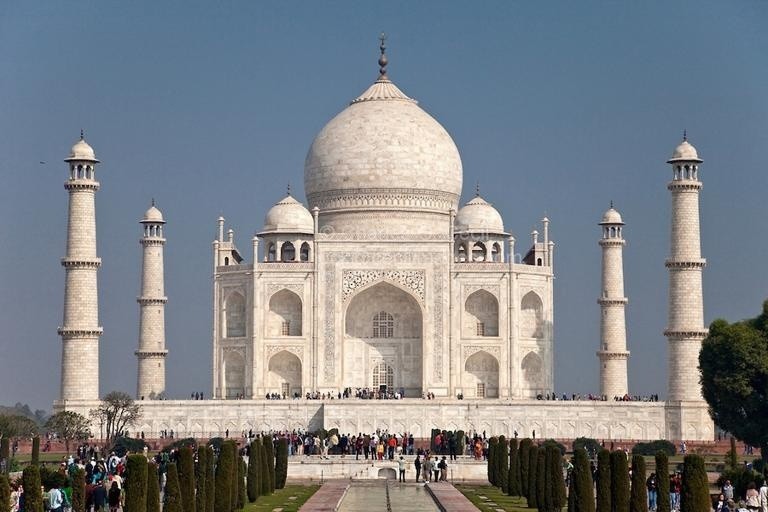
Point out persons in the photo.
[431,392,435,399]
[433,456,440,482]
[191,392,195,399]
[196,392,199,399]
[236,393,239,400]
[0,429,178,512]
[460,393,463,399]
[240,428,414,460]
[414,456,422,482]
[423,458,432,481]
[441,456,448,482]
[457,393,460,400]
[426,391,432,399]
[265,386,405,400]
[200,392,203,400]
[397,456,406,483]
[536,392,659,402]
[422,457,434,483]
[434,430,490,461]
[240,394,244,400]
[562,432,768,512]
[226,429,229,437]
